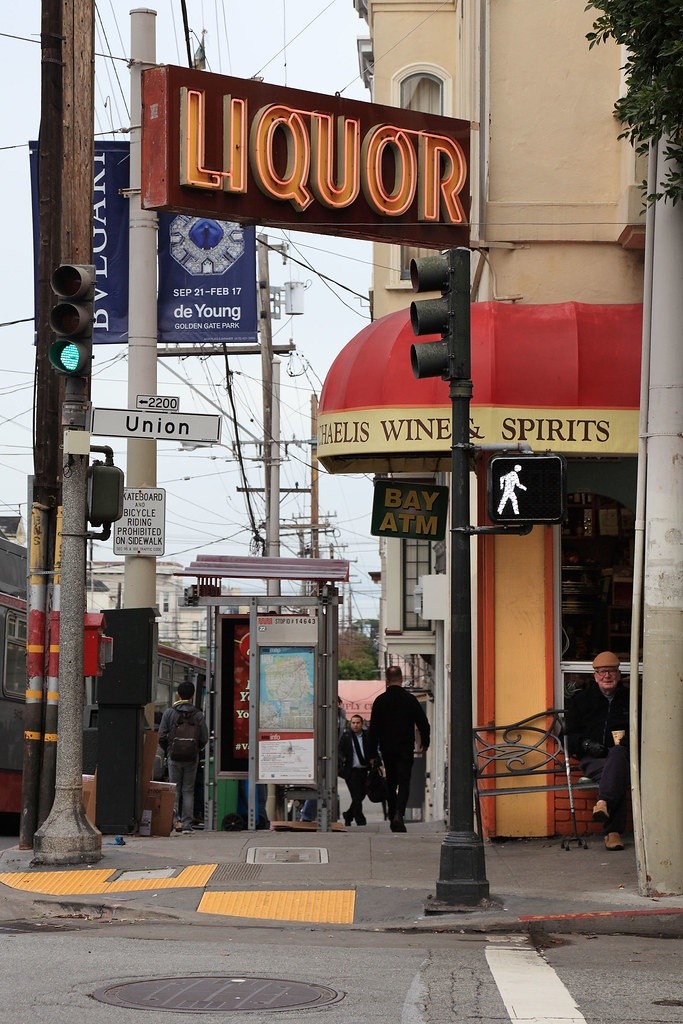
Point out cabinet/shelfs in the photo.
[561,493,643,663]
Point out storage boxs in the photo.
[80,731,177,838]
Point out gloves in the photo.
[582,739,609,758]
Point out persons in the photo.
[159,680,208,831]
[566,650,641,850]
[369,666,431,832]
[338,714,368,828]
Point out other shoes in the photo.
[605,832,624,850]
[389,813,406,832]
[594,804,609,823]
[176,822,182,831]
[181,823,194,833]
[342,812,352,827]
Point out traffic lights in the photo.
[48,263,98,379]
[407,250,457,383]
[489,453,567,524]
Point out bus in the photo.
[0,591,214,815]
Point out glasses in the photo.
[595,670,618,675]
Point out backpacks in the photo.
[168,707,201,763]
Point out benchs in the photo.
[471,710,600,845]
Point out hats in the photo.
[592,652,620,668]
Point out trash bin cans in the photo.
[201,758,268,833]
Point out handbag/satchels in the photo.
[367,765,388,803]
